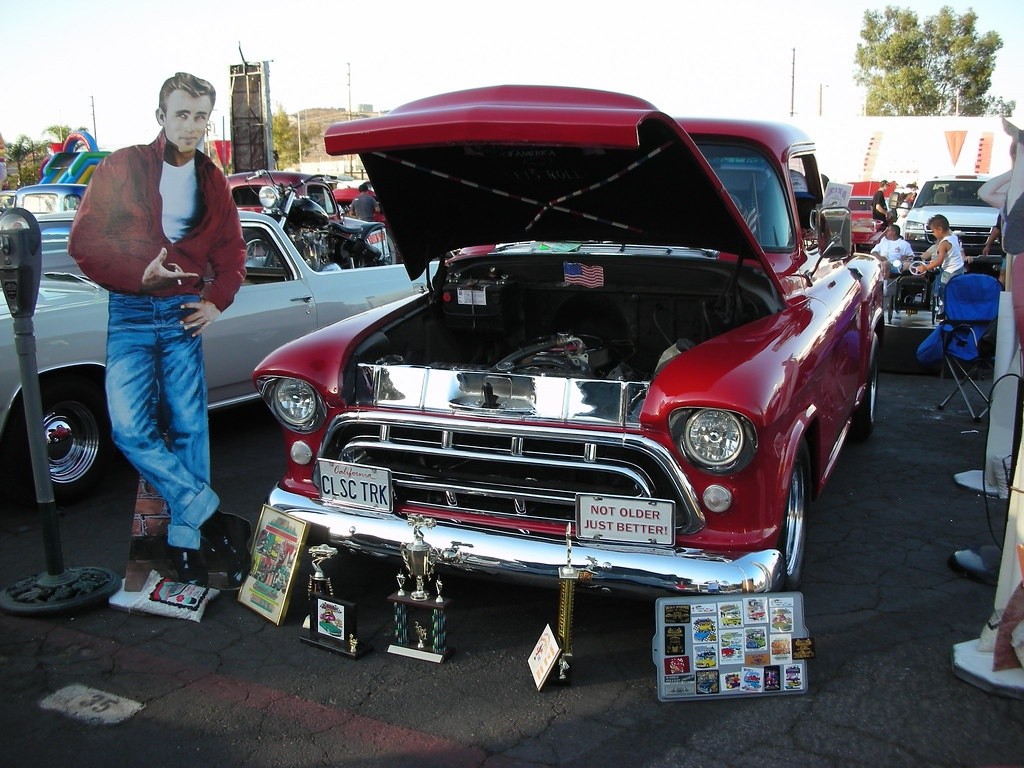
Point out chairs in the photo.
[915,273,1004,424]
[933,191,953,205]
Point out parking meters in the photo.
[0,207,122,615]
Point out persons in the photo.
[873,180,889,232]
[983,213,1006,283]
[350,184,380,222]
[916,214,973,322]
[907,186,917,209]
[870,224,915,274]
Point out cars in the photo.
[0,166,442,499]
[253,83,886,603]
[841,180,899,244]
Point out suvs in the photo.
[903,175,1004,268]
